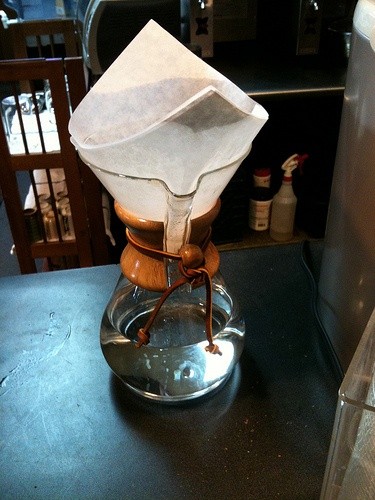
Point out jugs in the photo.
[78,143,252,402]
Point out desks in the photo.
[0,238,342,500]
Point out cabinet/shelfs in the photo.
[0,19,111,275]
[203,38,347,252]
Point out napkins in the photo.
[67,19,270,223]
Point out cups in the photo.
[22,190,76,243]
[1,75,73,154]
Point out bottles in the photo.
[249,169,273,241]
[320,308,375,500]
[270,154,308,241]
